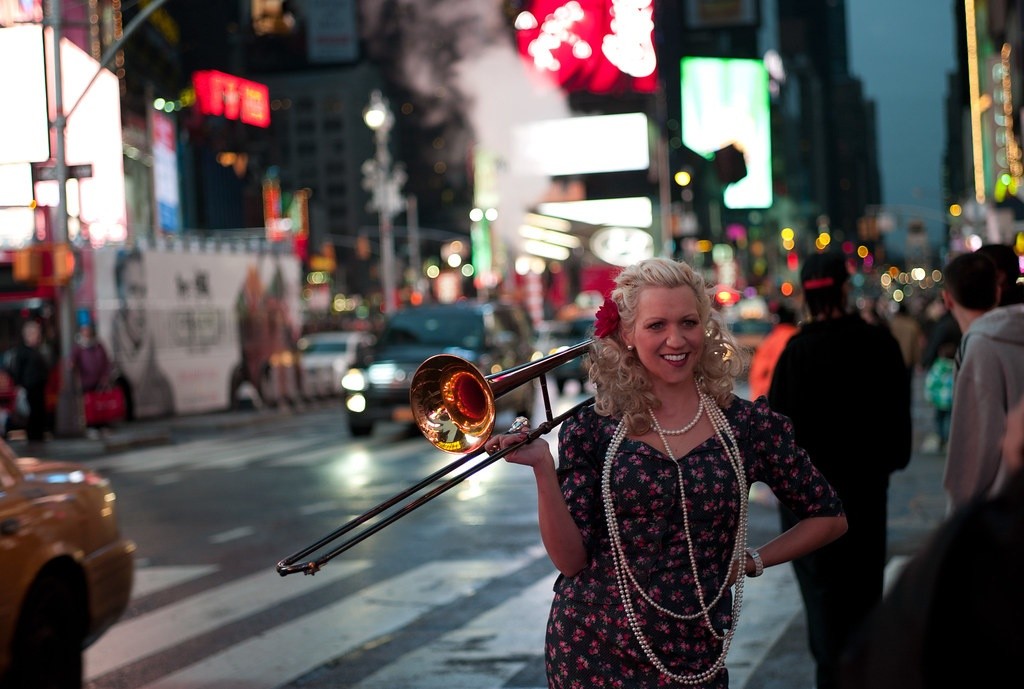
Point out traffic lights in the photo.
[713,285,735,309]
[504,0,657,117]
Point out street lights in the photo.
[363,92,408,310]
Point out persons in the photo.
[749,247,1024,689]
[483,257,848,689]
[7,319,51,449]
[108,249,176,415]
[67,323,112,439]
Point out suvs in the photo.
[549,315,595,393]
[342,299,541,438]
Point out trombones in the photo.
[273,339,595,577]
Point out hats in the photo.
[801,254,844,299]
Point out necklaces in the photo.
[602,379,749,684]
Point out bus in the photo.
[0,235,305,438]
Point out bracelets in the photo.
[745,547,764,578]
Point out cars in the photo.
[302,333,366,368]
[0,436,136,689]
